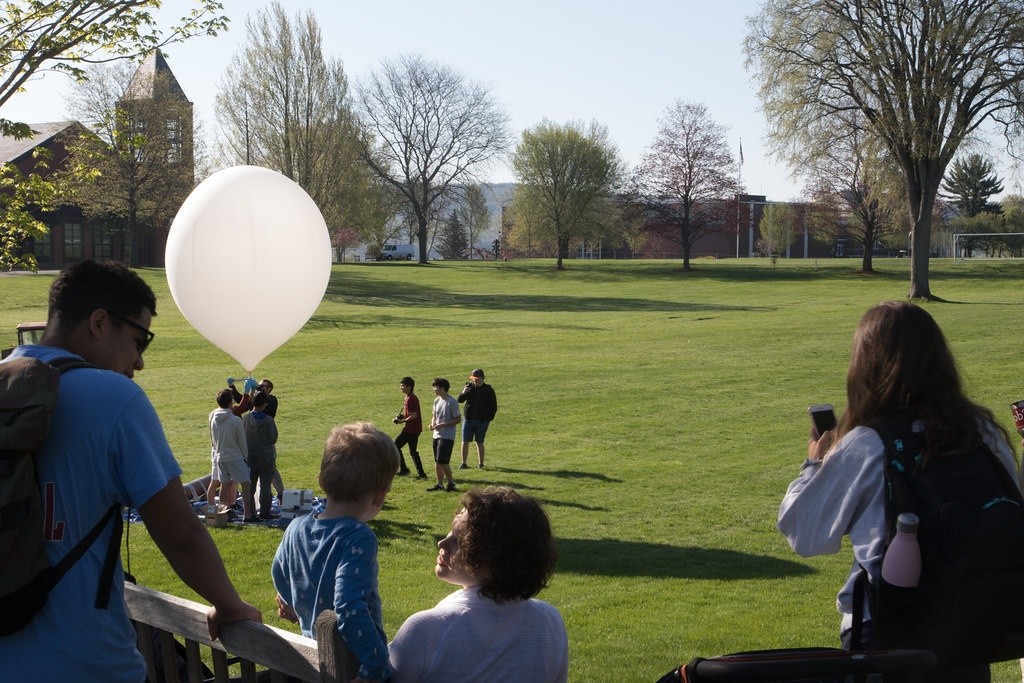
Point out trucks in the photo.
[380,243,415,261]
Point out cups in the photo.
[1010,399,1024,428]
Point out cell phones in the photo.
[808,403,837,437]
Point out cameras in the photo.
[393,414,405,424]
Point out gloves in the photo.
[228,378,235,385]
[244,377,258,394]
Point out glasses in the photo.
[260,384,267,387]
[89,309,155,355]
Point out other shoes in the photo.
[205,502,279,522]
[277,490,283,505]
[396,468,410,476]
[427,485,444,491]
[413,473,426,478]
[460,464,467,469]
[478,464,484,468]
[445,484,455,491]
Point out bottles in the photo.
[881,512,922,588]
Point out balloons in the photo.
[165,165,332,373]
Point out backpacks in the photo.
[857,405,1024,663]
[0,347,123,638]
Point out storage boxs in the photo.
[281,489,313,519]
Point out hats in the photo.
[469,369,484,380]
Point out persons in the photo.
[270,421,401,683]
[392,377,427,479]
[775,300,1024,683]
[387,486,569,683]
[426,377,461,492]
[207,389,234,505]
[242,392,280,519]
[0,259,263,683]
[457,368,497,469]
[210,391,264,522]
[227,377,284,505]
